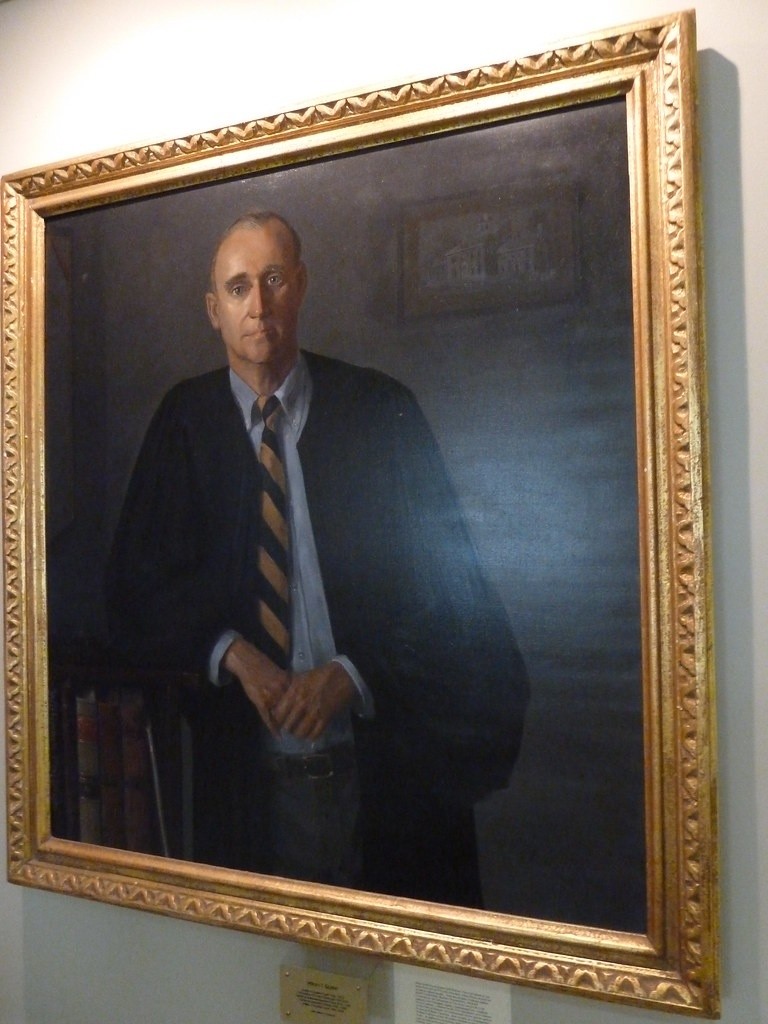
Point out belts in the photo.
[263,743,357,779]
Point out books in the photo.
[55,680,147,851]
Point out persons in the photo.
[104,216,531,909]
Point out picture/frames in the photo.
[2,8,726,1016]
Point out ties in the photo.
[255,395,293,668]
